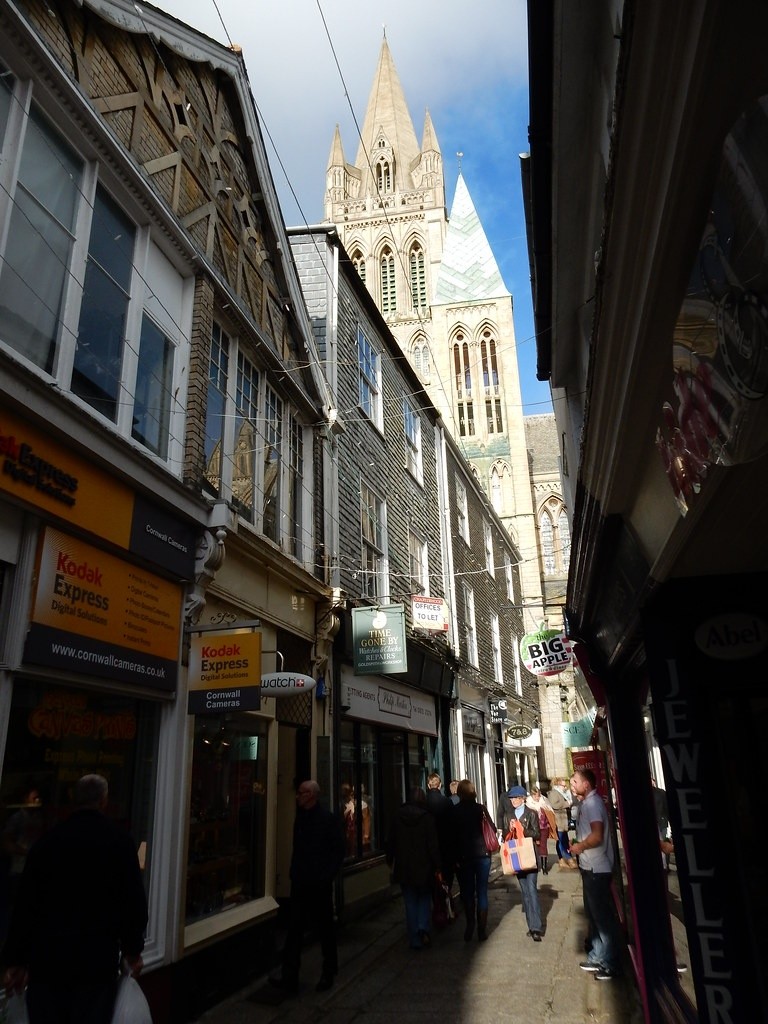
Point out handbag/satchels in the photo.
[500,820,537,876]
[432,872,458,928]
[111,959,153,1024]
[480,805,500,852]
[0,987,30,1024]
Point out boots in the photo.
[541,857,548,875]
[477,904,489,940]
[464,898,475,941]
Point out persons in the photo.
[567,768,624,981]
[389,784,441,953]
[502,786,545,942]
[426,773,460,922]
[3,774,149,1024]
[526,777,579,875]
[280,780,346,995]
[661,819,687,974]
[5,789,48,877]
[449,780,497,943]
[186,841,224,921]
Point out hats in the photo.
[508,786,527,797]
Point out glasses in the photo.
[297,791,311,795]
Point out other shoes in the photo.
[594,969,612,979]
[677,964,688,972]
[315,966,339,992]
[558,858,579,869]
[580,962,602,971]
[527,930,541,941]
[408,929,432,949]
